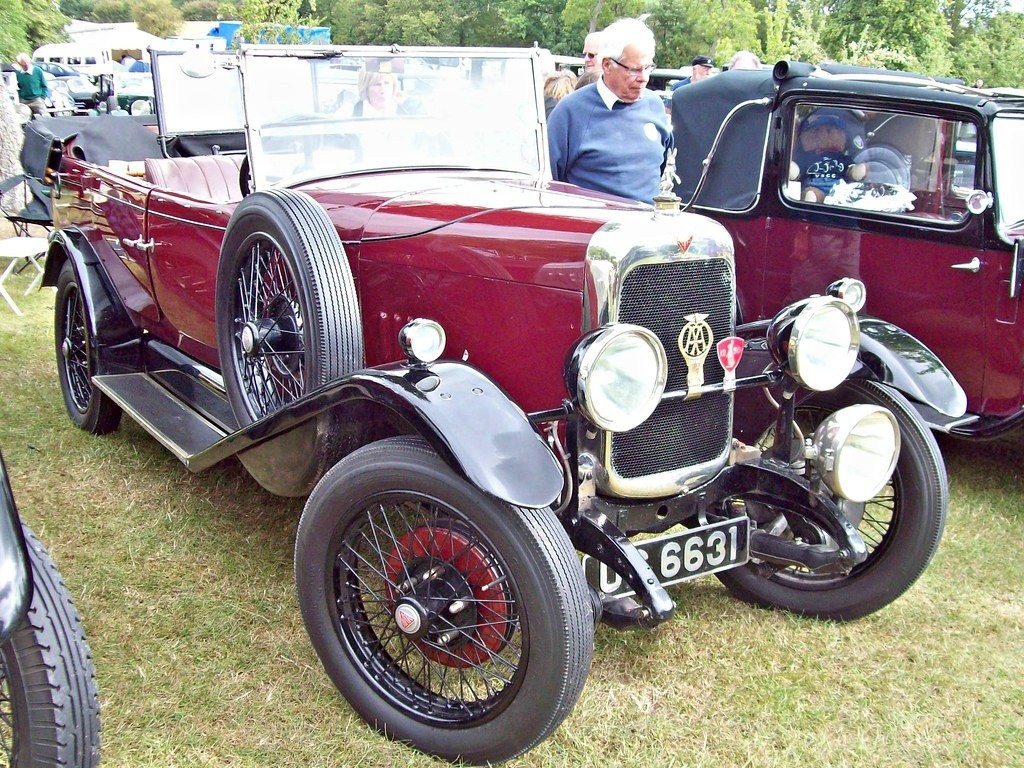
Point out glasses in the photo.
[582,52,598,58]
[608,57,657,76]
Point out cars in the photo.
[668,59,1024,437]
[19,39,967,766]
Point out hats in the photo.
[692,55,714,68]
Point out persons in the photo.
[544,18,763,209]
[15,53,52,129]
[352,63,407,117]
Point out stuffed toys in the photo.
[789,107,868,203]
